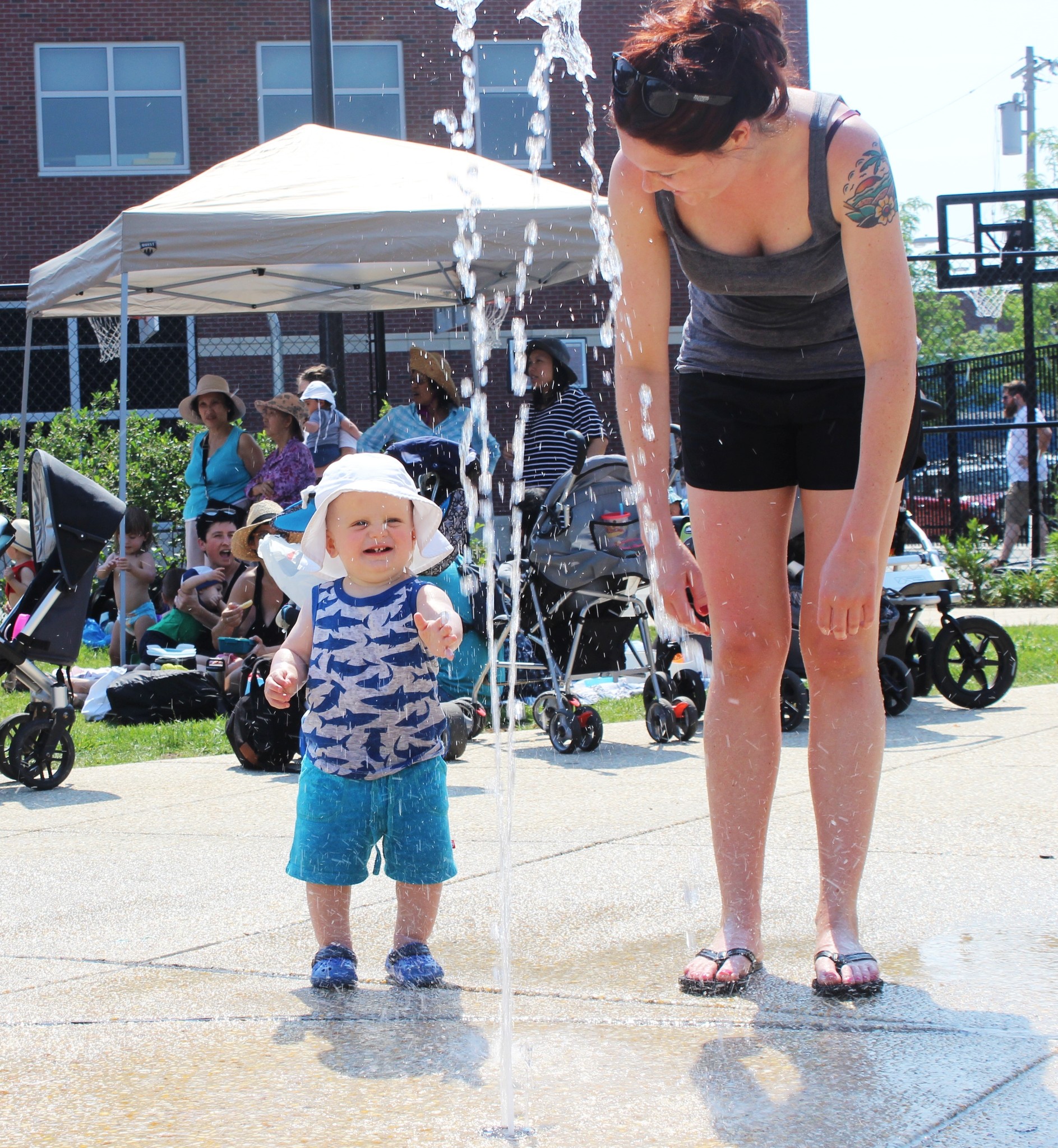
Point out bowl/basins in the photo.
[217,637,255,654]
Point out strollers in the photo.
[640,389,1020,733]
[445,422,705,751]
[0,447,141,790]
[227,434,480,771]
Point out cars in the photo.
[905,464,1008,538]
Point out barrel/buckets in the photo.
[154,656,197,671]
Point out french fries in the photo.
[237,599,253,609]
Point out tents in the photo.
[19,120,610,671]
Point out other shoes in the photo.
[385,942,443,990]
[307,945,360,990]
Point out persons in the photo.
[357,347,501,476]
[265,452,464,996]
[98,503,157,667]
[3,518,34,689]
[70,508,250,709]
[605,1,921,994]
[178,375,266,569]
[983,380,1052,572]
[137,565,226,667]
[295,365,358,457]
[212,502,300,696]
[245,392,314,511]
[504,337,608,488]
[157,567,188,624]
[299,379,362,486]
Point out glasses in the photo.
[202,509,237,517]
[250,530,290,543]
[610,50,734,119]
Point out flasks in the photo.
[205,657,225,691]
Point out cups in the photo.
[601,511,631,548]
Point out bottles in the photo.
[12,613,30,641]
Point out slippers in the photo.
[678,948,763,1000]
[812,951,882,1000]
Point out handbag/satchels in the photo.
[203,496,246,530]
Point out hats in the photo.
[525,338,578,387]
[178,374,246,426]
[181,566,227,592]
[8,518,34,556]
[253,392,311,443]
[408,346,462,410]
[300,380,336,412]
[232,499,304,562]
[297,452,455,580]
